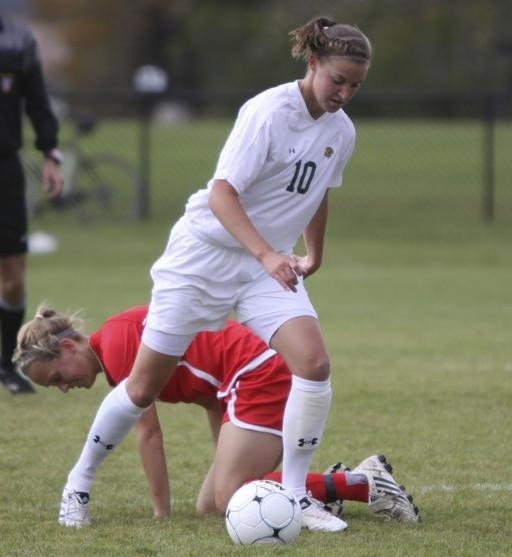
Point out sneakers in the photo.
[0,369,35,393]
[319,462,350,517]
[58,485,93,529]
[351,453,423,524]
[295,494,348,533]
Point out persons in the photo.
[58,18,371,532]
[13,305,423,523]
[0,10,74,389]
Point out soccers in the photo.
[225,480,303,546]
